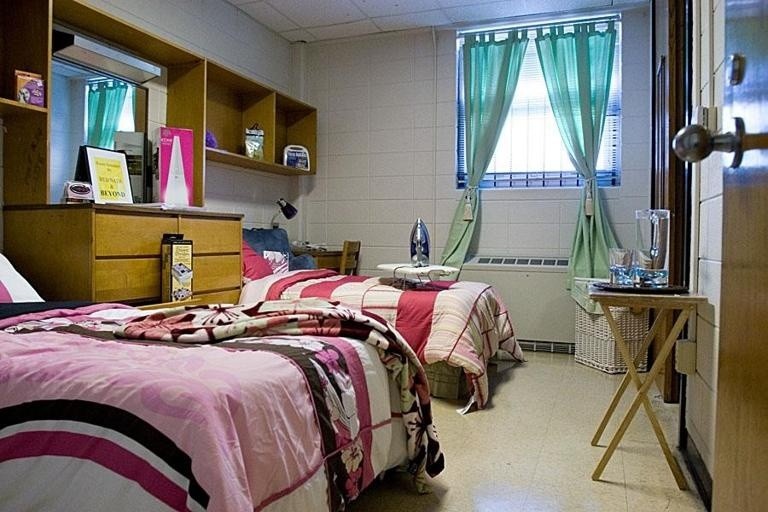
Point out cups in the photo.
[634,209,671,290]
[606,247,635,288]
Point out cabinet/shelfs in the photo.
[0,0,208,207]
[205,58,318,176]
[0,204,245,312]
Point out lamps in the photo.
[271,197,298,229]
[52,29,162,87]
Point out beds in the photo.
[0,252,450,511]
[239,228,528,414]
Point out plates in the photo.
[594,281,690,295]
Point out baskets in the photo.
[574,306,650,376]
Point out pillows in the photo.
[263,251,289,274]
[290,252,318,271]
[243,237,274,283]
[243,228,290,253]
[0,252,45,304]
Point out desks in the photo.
[290,247,342,270]
[589,285,708,491]
[376,261,460,292]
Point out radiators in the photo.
[457,256,581,355]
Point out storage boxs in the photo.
[112,130,145,204]
[148,126,194,210]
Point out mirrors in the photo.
[51,56,149,203]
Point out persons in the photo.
[18,87,31,104]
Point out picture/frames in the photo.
[83,144,136,205]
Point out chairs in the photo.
[339,239,362,276]
